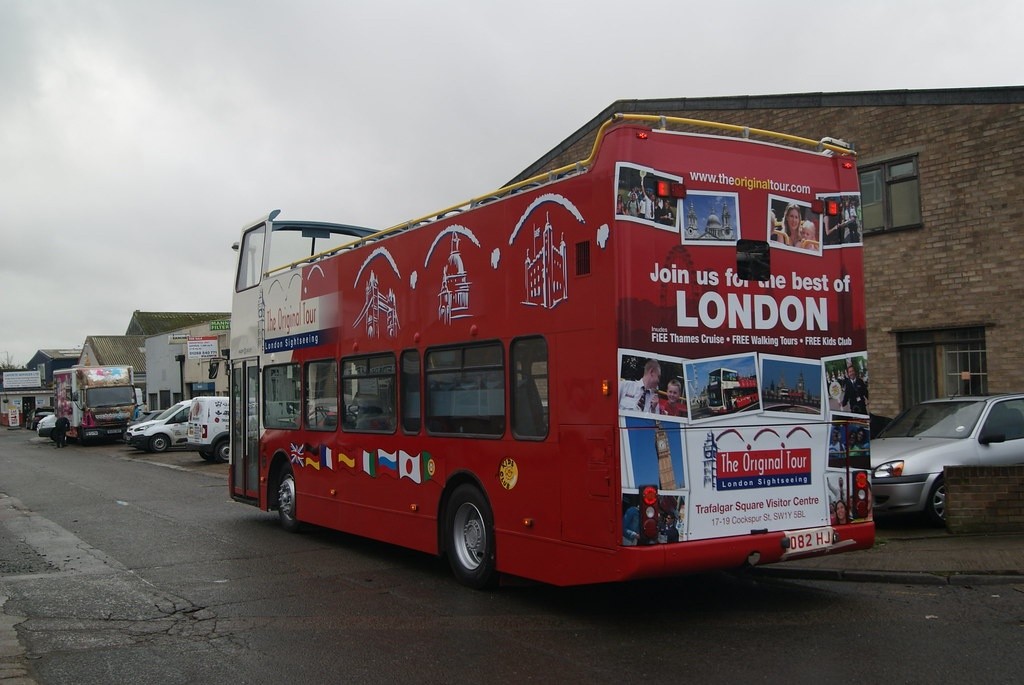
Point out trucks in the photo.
[53,366,137,444]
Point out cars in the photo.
[869,393,1024,522]
[126,411,164,429]
[36,414,58,443]
[26,406,55,430]
[187,397,261,461]
[291,394,365,430]
[870,411,894,442]
[126,398,193,451]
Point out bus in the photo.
[780,386,804,398]
[708,366,758,414]
[229,112,875,588]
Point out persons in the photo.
[843,430,860,460]
[851,481,872,524]
[863,420,870,446]
[853,424,869,458]
[351,397,377,431]
[847,493,854,520]
[839,365,869,415]
[616,170,675,227]
[848,201,860,230]
[618,357,663,416]
[844,199,850,225]
[824,425,846,461]
[622,500,644,546]
[662,513,681,545]
[779,203,809,249]
[791,220,820,251]
[54,411,73,449]
[770,208,787,248]
[835,500,852,523]
[822,200,842,247]
[656,377,689,421]
[832,499,837,510]
[829,503,836,524]
[825,472,852,507]
